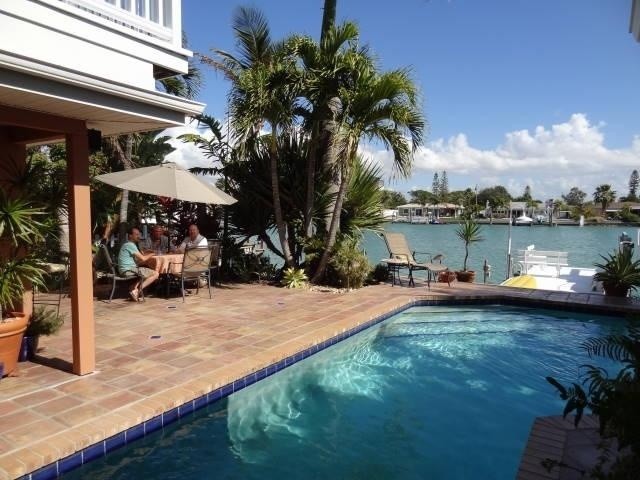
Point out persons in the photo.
[117,227,159,303]
[176,224,208,295]
[145,226,176,292]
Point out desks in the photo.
[32,263,66,317]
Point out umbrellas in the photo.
[93,161,239,296]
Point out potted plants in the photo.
[0,186,69,380]
[453,216,481,283]
[17,304,65,362]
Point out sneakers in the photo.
[128,289,138,304]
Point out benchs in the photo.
[517,250,568,277]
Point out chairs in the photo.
[99,238,223,303]
[379,232,451,289]
